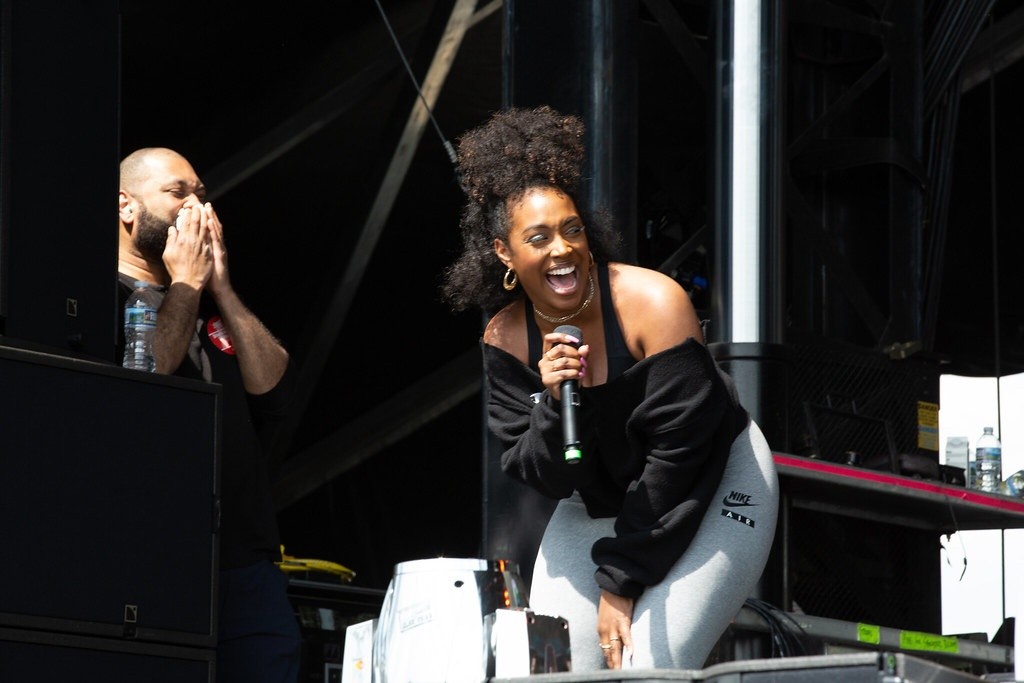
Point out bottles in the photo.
[1000,478,1024,495]
[122,282,158,372]
[975,427,1001,493]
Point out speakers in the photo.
[0,0,222,683]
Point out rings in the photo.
[609,636,620,641]
[599,643,612,649]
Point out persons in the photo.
[118,146,306,683]
[437,105,786,683]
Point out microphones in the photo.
[552,326,584,463]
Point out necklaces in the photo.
[532,270,596,324]
